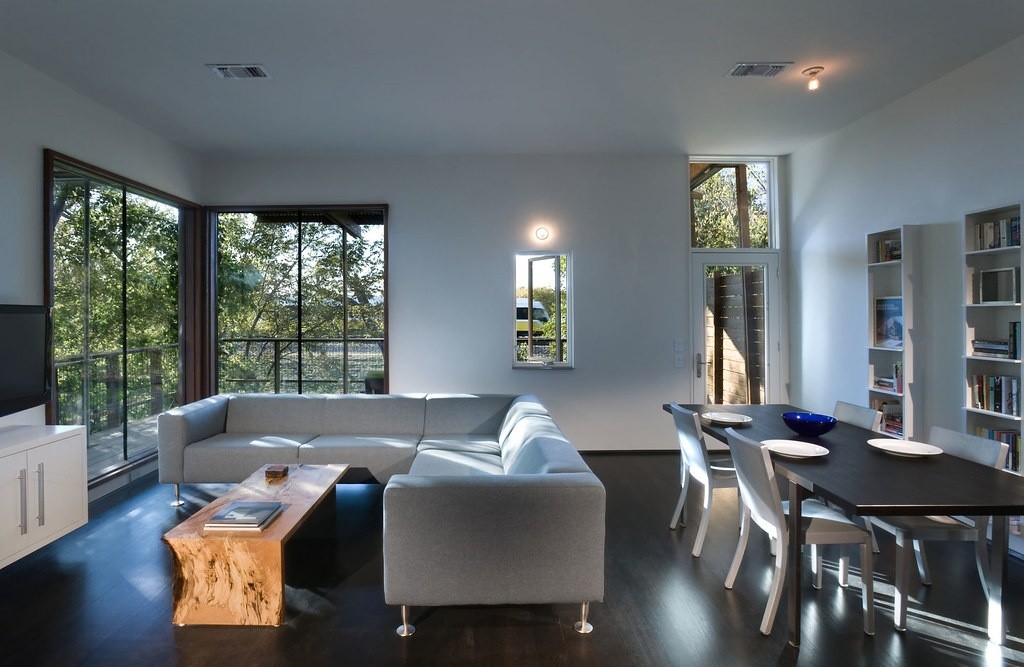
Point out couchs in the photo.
[157,392,606,638]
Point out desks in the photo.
[663,404,1024,647]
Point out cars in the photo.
[516,297,549,338]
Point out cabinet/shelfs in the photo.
[0,424,88,570]
[864,225,923,442]
[963,200,1024,555]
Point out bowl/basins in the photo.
[782,412,837,437]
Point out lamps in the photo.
[802,66,825,92]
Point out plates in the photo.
[701,412,754,425]
[866,438,944,457]
[760,439,830,458]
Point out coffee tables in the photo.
[160,463,350,627]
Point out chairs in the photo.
[669,401,740,557]
[839,425,1008,631]
[725,427,874,634]
[805,401,883,553]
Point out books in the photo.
[874,240,902,262]
[874,361,903,393]
[973,425,1021,473]
[975,216,1021,251]
[204,501,282,527]
[870,398,904,436]
[972,374,1021,416]
[202,501,284,533]
[971,321,1021,360]
